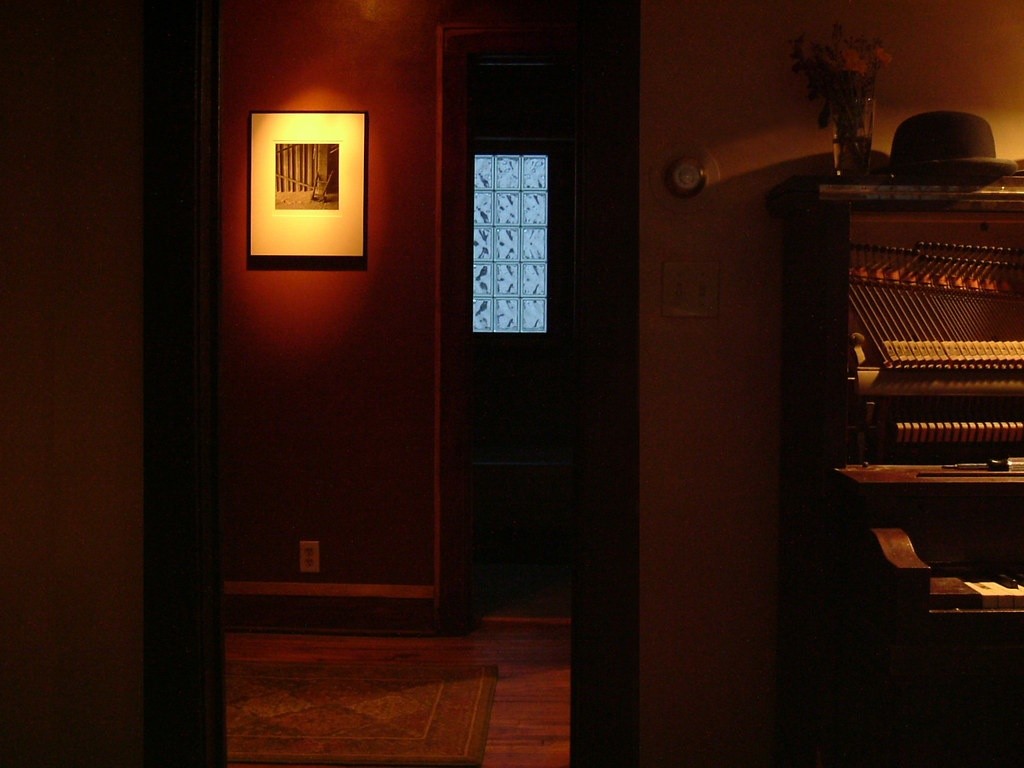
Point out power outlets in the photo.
[299,541,321,573]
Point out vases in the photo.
[830,96,876,185]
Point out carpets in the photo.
[216,657,499,768]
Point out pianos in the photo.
[763,170,1024,768]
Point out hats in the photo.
[876,111,1018,175]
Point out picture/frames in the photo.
[246,109,370,261]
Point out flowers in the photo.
[787,21,894,159]
